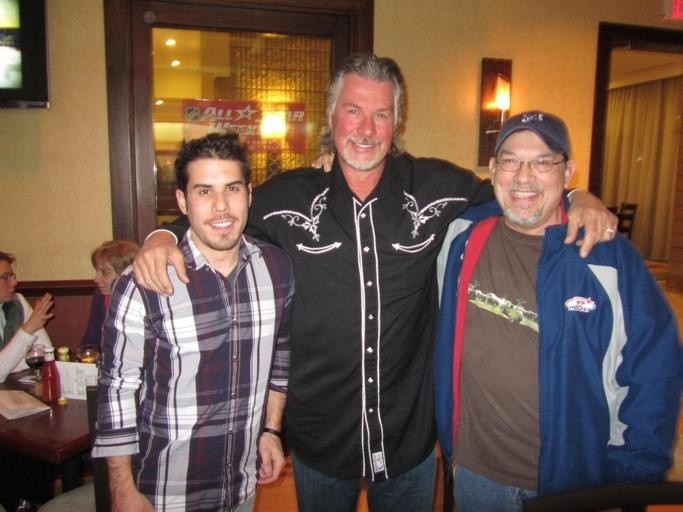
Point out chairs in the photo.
[38,385,113,512]
[616,201,638,240]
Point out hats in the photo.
[495,113,570,159]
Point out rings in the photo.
[605,227,615,234]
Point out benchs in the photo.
[13,280,101,354]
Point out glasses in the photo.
[496,158,564,174]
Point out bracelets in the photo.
[262,425,284,439]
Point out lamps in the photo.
[477,57,513,167]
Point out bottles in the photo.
[57,346,70,362]
[41,345,60,403]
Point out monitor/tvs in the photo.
[0,0,51,108]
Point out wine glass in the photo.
[25,343,46,381]
[74,343,98,364]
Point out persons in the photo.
[0,249,56,384]
[434,103,683,511]
[80,238,142,352]
[130,49,626,512]
[91,130,298,512]
